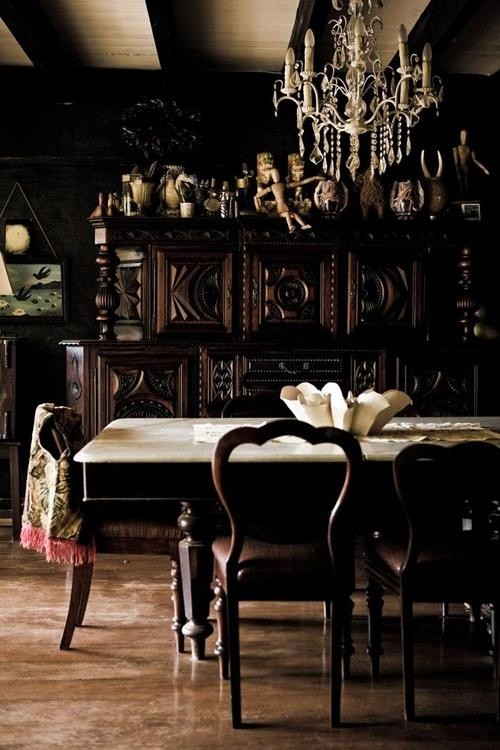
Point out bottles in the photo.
[394,181,419,220]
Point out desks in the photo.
[74,413,500,506]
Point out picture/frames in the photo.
[0,257,69,324]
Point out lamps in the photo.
[270,0,447,182]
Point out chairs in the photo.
[209,405,363,672]
[18,397,193,655]
[0,340,22,547]
[362,446,500,669]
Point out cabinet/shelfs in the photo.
[60,214,500,442]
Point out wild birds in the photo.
[13,285,33,302]
[32,265,52,281]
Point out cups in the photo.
[179,202,194,218]
